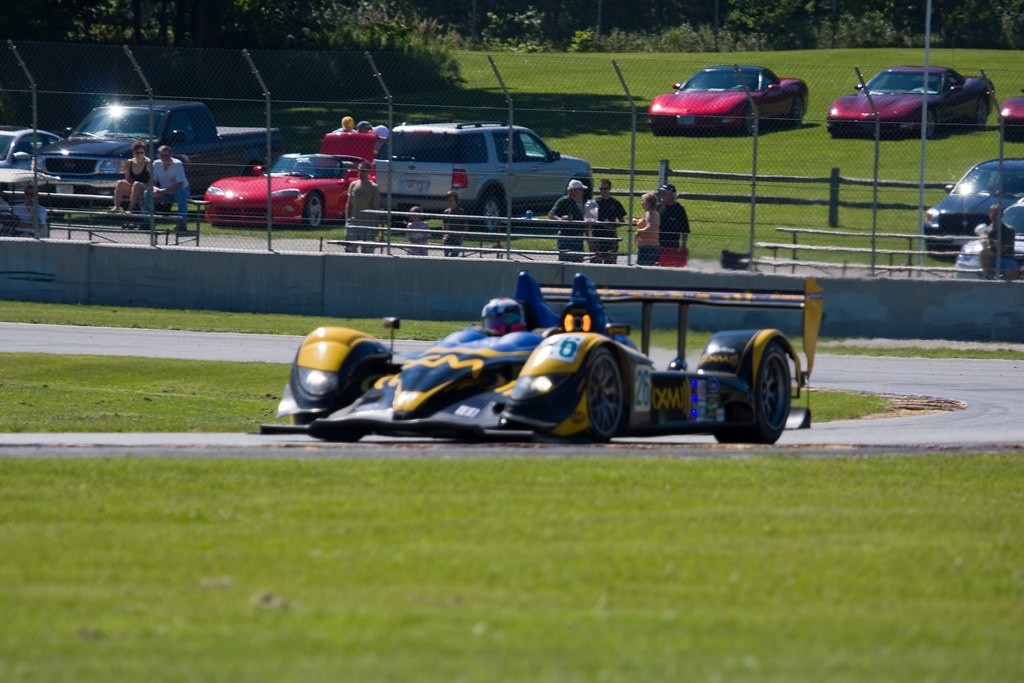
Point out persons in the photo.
[584,179,627,264]
[139,145,191,231]
[548,177,587,263]
[979,205,1018,281]
[333,116,389,151]
[633,184,690,268]
[405,206,432,255]
[474,297,526,336]
[344,162,379,254]
[0,185,48,238]
[443,190,468,257]
[110,141,152,214]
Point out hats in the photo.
[341,116,354,128]
[567,179,588,190]
[357,121,373,130]
[372,125,389,139]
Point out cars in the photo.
[370,120,594,235]
[953,198,1024,280]
[924,157,1024,261]
[0,125,66,221]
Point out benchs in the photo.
[0,189,211,247]
[738,226,1024,280]
[327,208,635,264]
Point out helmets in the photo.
[482,298,527,336]
[657,184,676,194]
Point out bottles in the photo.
[583,199,598,221]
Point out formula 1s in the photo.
[259,270,824,445]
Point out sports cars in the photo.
[826,65,995,139]
[998,88,1024,143]
[204,132,378,231]
[647,65,809,137]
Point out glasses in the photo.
[654,193,667,197]
[599,187,609,191]
[356,169,367,172]
[133,151,143,154]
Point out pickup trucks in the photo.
[31,99,285,220]
